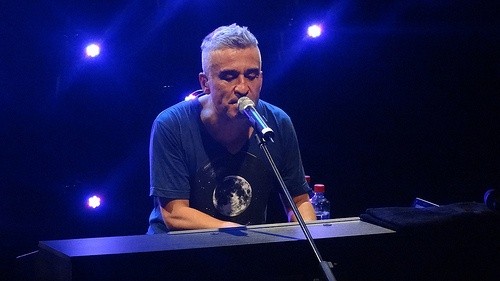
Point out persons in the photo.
[146,23,317,236]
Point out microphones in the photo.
[237,96,275,143]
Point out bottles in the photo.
[304,175,315,201]
[309,183,331,220]
